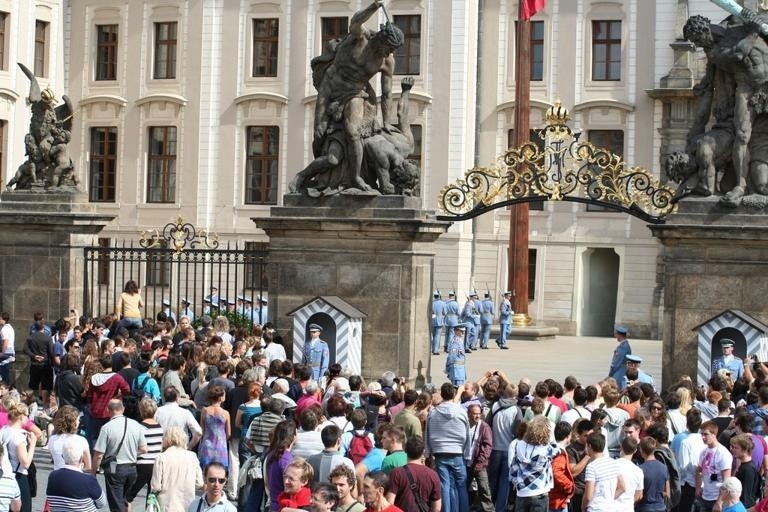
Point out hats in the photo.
[453,323,466,331]
[309,323,323,332]
[434,291,512,297]
[720,339,735,348]
[615,326,628,335]
[161,285,267,307]
[625,354,642,363]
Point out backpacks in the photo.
[123,376,151,421]
[343,430,373,465]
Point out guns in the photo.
[435,281,512,315]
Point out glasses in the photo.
[207,477,227,484]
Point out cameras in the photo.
[393,378,400,383]
[79,428,89,438]
[71,310,74,312]
[454,385,460,389]
[493,372,498,375]
[751,355,757,360]
[726,373,730,377]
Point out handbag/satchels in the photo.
[100,455,117,475]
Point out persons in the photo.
[47,129,73,191]
[662,117,768,195]
[1,278,470,512]
[290,76,419,199]
[310,1,405,191]
[461,326,767,512]
[6,148,43,194]
[432,289,514,355]
[682,7,768,206]
